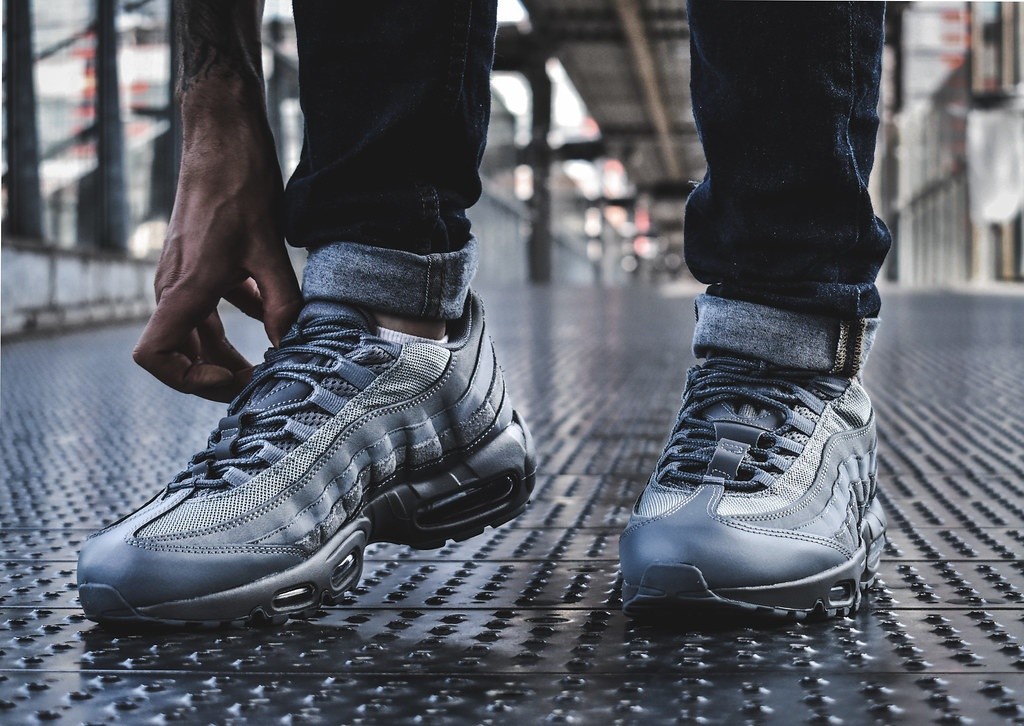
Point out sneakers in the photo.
[77,286,537,625]
[617,353,888,618]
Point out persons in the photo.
[78,2,895,630]
